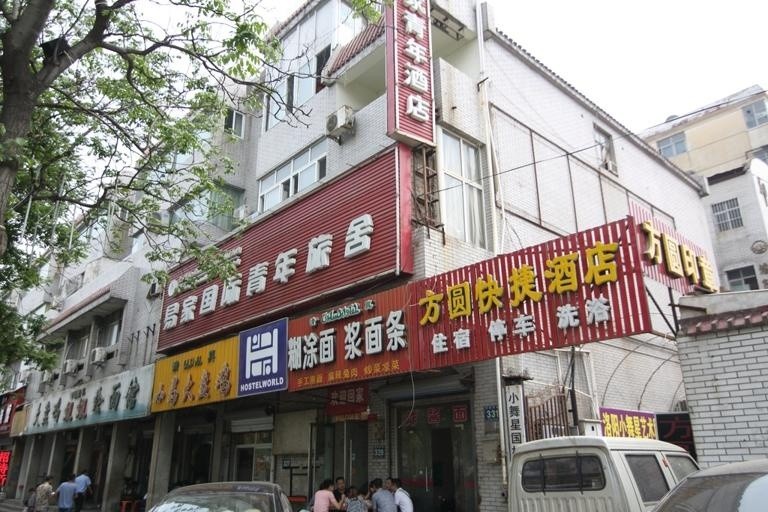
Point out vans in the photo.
[507,436,702,512]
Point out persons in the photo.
[308,477,414,512]
[23,468,93,512]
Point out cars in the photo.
[650,458,768,512]
[148,481,294,512]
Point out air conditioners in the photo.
[324,104,355,144]
[40,346,107,384]
